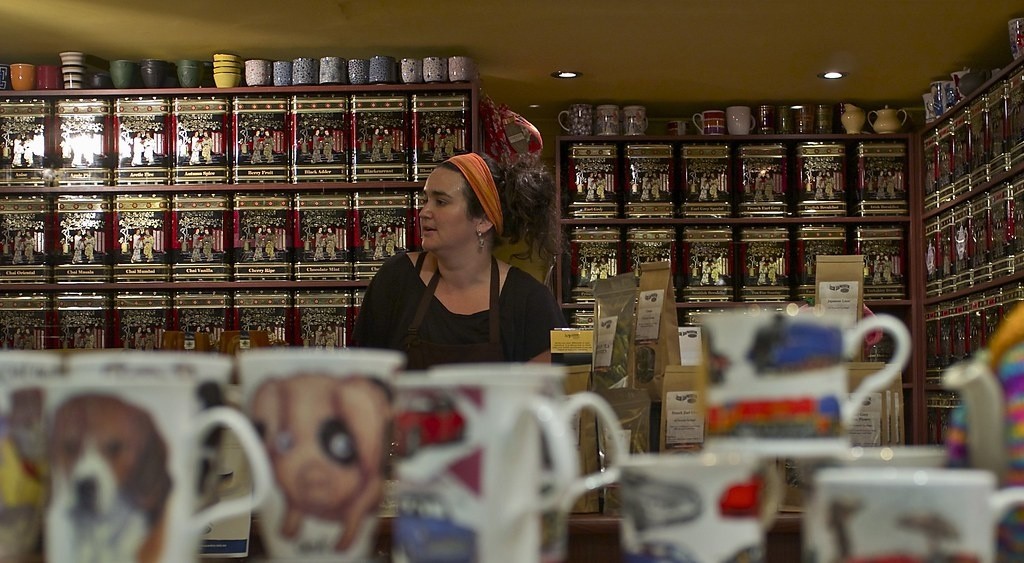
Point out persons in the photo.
[348,152,567,374]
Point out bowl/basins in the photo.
[107,54,242,89]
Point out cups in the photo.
[66,351,232,520]
[390,373,579,563]
[726,106,755,135]
[245,56,476,86]
[698,309,912,457]
[810,468,1024,563]
[597,105,619,135]
[237,349,407,563]
[0,350,76,563]
[757,106,908,133]
[559,104,593,136]
[41,376,273,563]
[427,362,628,562]
[832,445,949,473]
[619,454,779,563]
[1008,17,1024,60]
[622,105,727,136]
[10,52,107,91]
[920,70,970,123]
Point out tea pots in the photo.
[939,301,1024,557]
